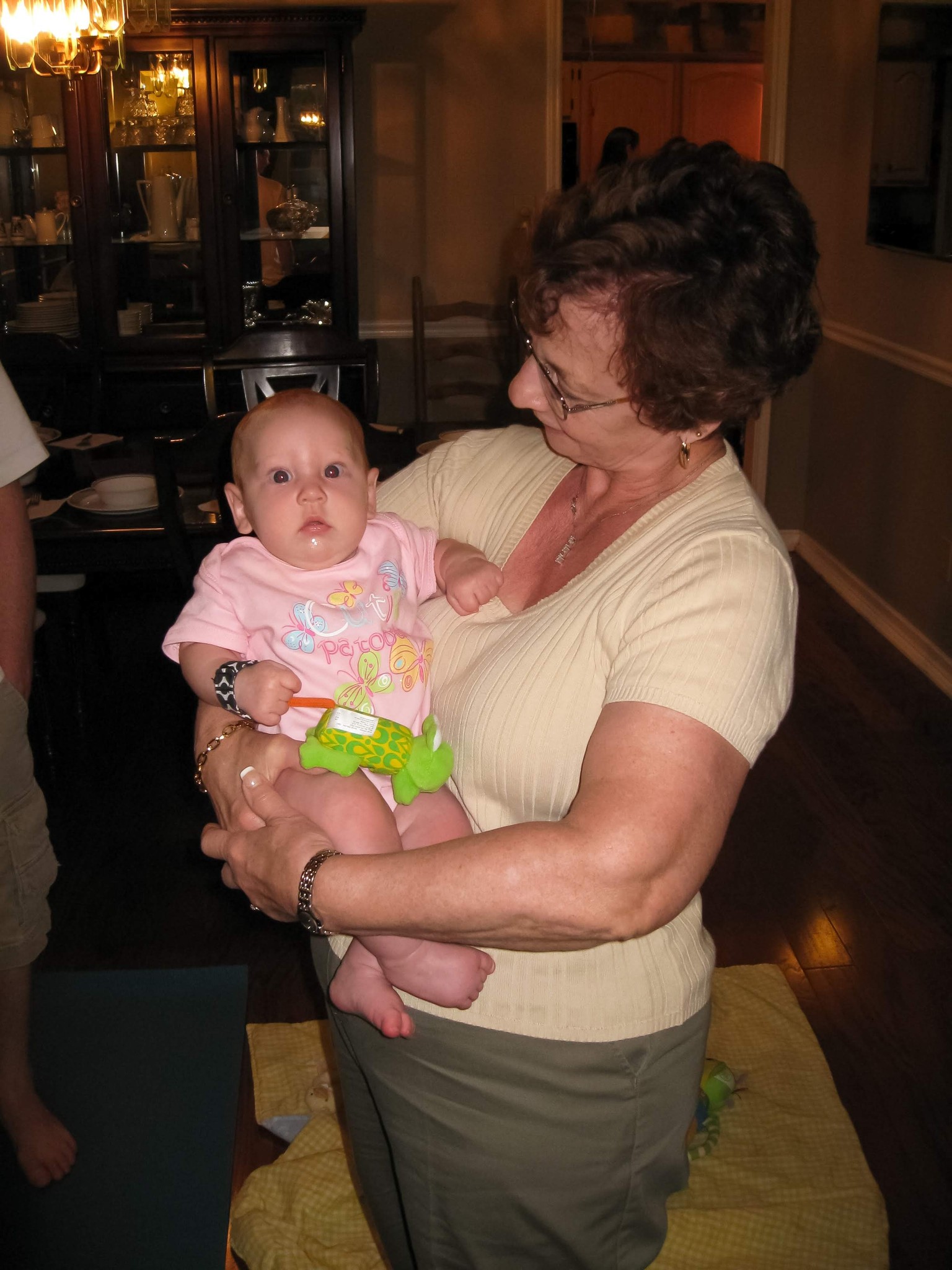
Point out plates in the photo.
[417,439,449,454]
[66,487,159,515]
[38,292,77,302]
[4,302,80,338]
[26,498,66,519]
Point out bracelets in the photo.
[211,660,260,722]
[193,720,255,793]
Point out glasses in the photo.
[509,299,643,422]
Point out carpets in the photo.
[1,964,249,1270]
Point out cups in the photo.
[130,303,151,325]
[185,217,199,227]
[118,309,141,336]
[31,113,56,148]
[186,227,199,241]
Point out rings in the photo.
[250,904,260,912]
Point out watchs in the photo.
[297,850,343,936]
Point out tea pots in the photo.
[25,207,65,244]
[136,168,186,240]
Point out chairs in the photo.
[413,274,522,429]
[201,323,379,424]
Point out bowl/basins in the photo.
[91,473,159,509]
[439,430,469,443]
[37,426,61,444]
[10,236,25,245]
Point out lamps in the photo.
[0,0,132,90]
[147,51,192,96]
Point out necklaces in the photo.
[554,446,725,564]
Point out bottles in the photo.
[266,186,316,239]
[4,216,22,238]
[273,97,290,142]
[292,183,320,233]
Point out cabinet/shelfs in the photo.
[561,61,764,188]
[0,2,380,430]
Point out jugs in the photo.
[244,107,264,142]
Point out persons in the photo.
[201,134,823,1270]
[0,356,78,1190]
[161,389,504,1038]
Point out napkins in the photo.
[27,497,68,520]
[49,432,125,451]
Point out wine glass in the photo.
[110,87,196,145]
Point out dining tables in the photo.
[22,419,538,785]
